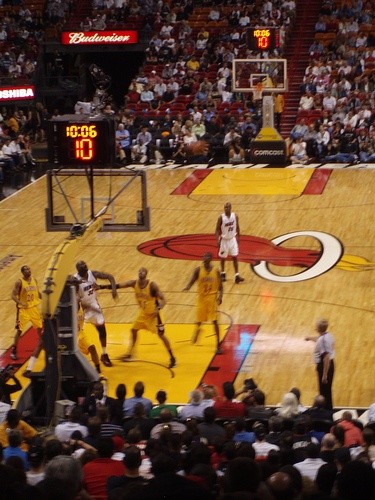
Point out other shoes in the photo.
[220,272,225,281]
[235,274,244,284]
[169,358,175,367]
[10,353,17,360]
[101,353,112,366]
[216,348,222,355]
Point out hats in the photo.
[320,434,340,453]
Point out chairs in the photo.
[126,8,263,117]
[296,0,375,125]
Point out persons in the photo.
[0,0,375,201]
[215,202,244,284]
[11,265,44,360]
[182,252,223,347]
[0,368,375,500]
[304,320,334,411]
[73,260,119,367]
[76,292,101,374]
[92,267,176,367]
[23,332,44,377]
